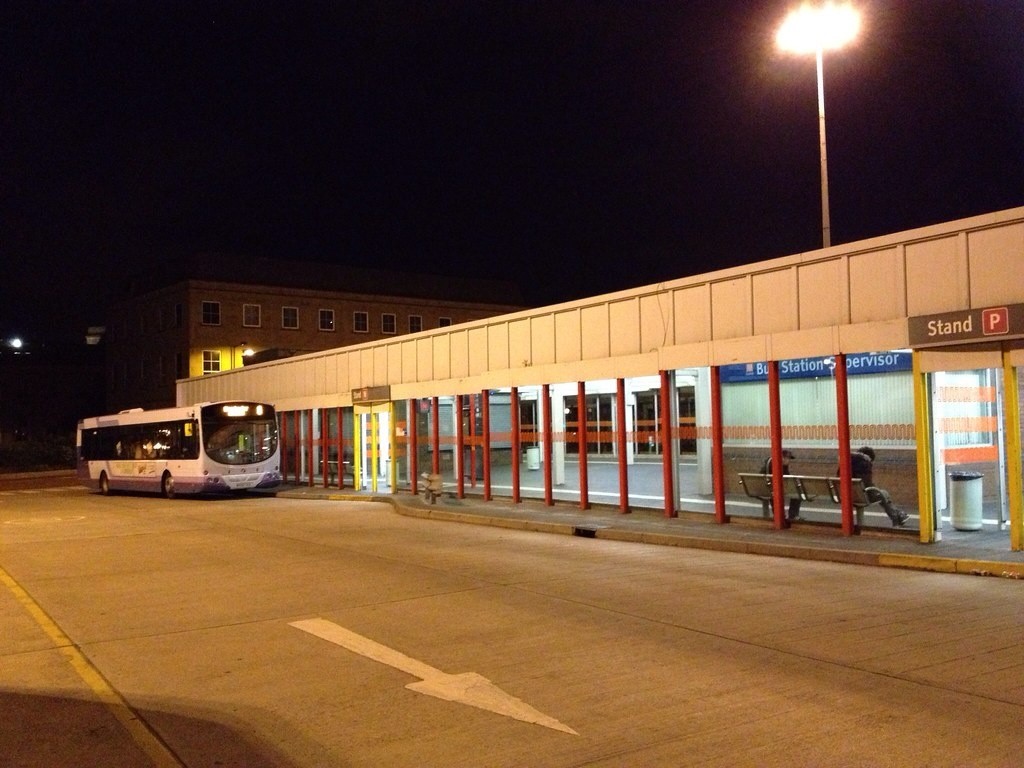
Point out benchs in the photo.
[737,472,891,536]
[319,460,363,486]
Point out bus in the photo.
[76,400,283,499]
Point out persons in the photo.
[759,449,806,521]
[836,446,912,527]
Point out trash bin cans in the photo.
[949,471,985,530]
[525,446,540,470]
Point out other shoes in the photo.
[893,515,909,526]
[788,514,803,521]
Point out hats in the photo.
[782,450,795,459]
